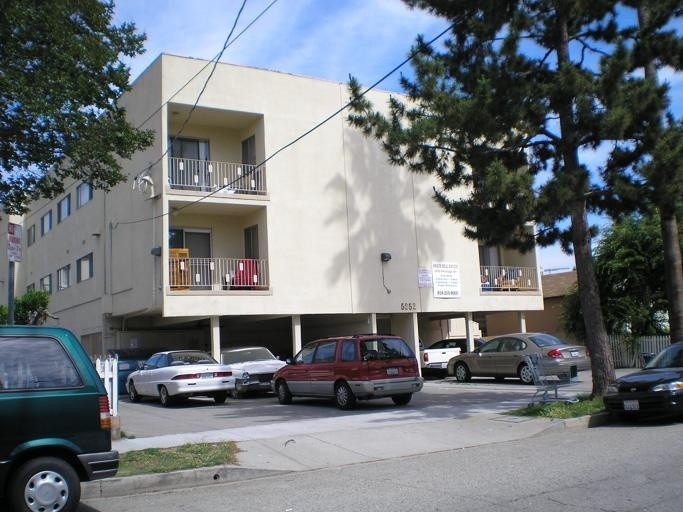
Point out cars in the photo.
[445,332,590,382]
[218,345,291,397]
[600,341,683,426]
[424,337,487,376]
[125,349,235,407]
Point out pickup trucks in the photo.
[418,337,462,373]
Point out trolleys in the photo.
[638,350,655,370]
[521,351,581,408]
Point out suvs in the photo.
[0,324,119,511]
[272,334,425,408]
